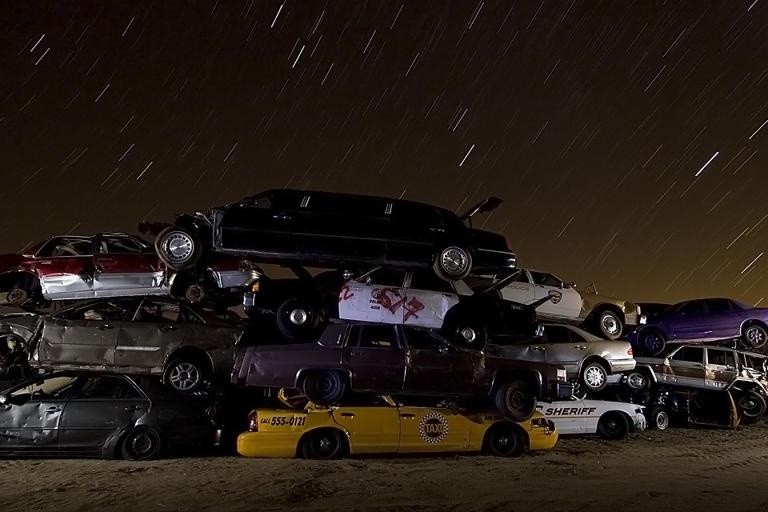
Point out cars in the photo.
[235,388,559,462]
[0,234,768,441]
[1,369,211,463]
[136,187,528,284]
